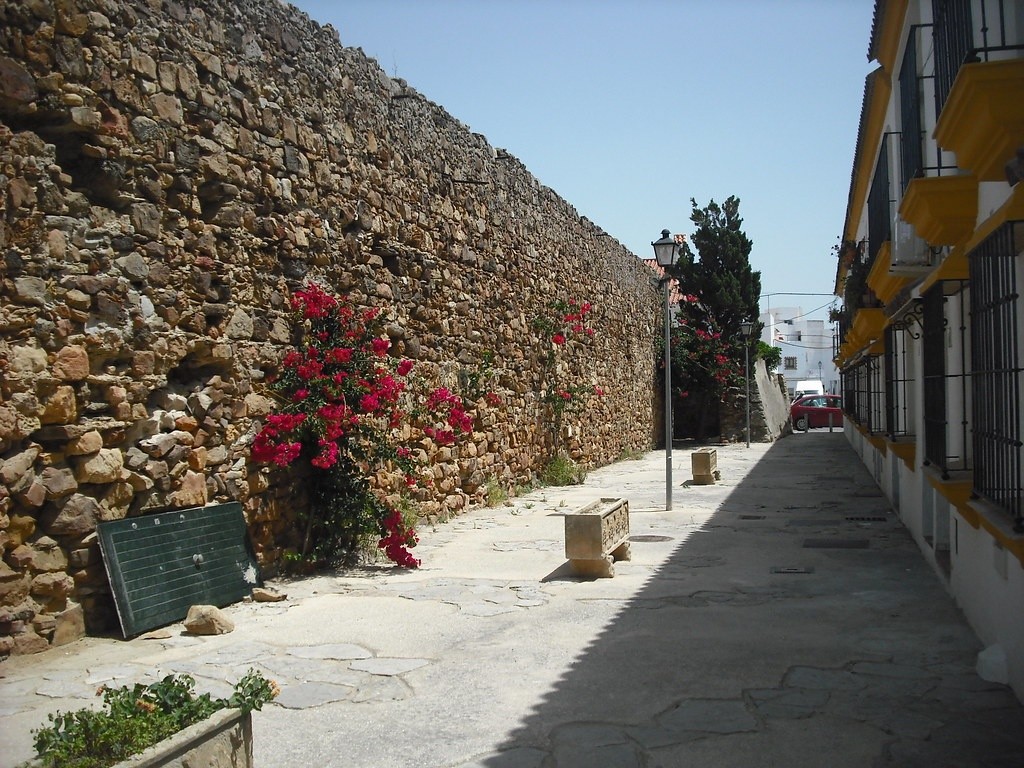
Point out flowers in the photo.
[32,672,279,768]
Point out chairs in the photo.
[813,400,819,406]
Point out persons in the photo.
[827,399,838,407]
[797,393,803,400]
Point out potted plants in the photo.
[830,238,878,341]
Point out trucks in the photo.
[788,380,824,406]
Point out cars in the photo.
[791,395,844,431]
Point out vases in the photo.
[17,697,253,768]
[692,447,717,475]
[564,497,630,560]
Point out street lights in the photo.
[817,360,823,381]
[651,227,680,511]
[739,320,754,450]
